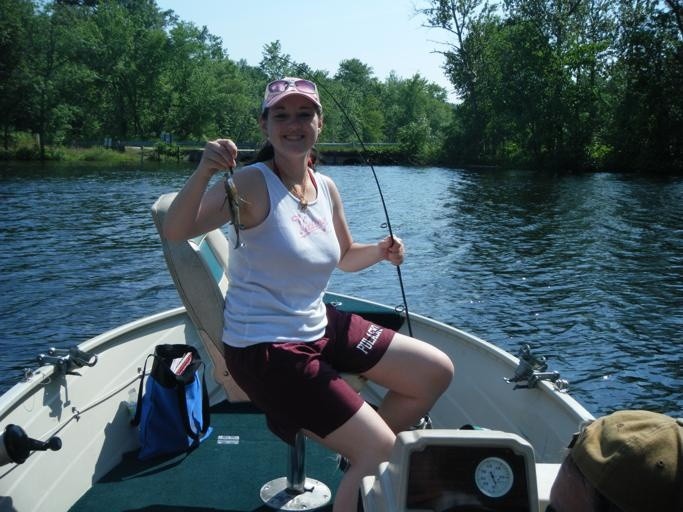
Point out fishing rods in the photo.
[257,48,433,431]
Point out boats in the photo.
[0,190,596,510]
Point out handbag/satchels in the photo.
[132,344,211,461]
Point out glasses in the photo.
[268,79,317,94]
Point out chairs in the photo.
[149,190,368,511]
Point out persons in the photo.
[543,408,682,511]
[160,76,455,512]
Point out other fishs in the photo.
[219,175,251,249]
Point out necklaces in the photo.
[275,162,309,211]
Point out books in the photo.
[169,351,194,375]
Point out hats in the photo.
[262,77,323,113]
[569,409,683,511]
